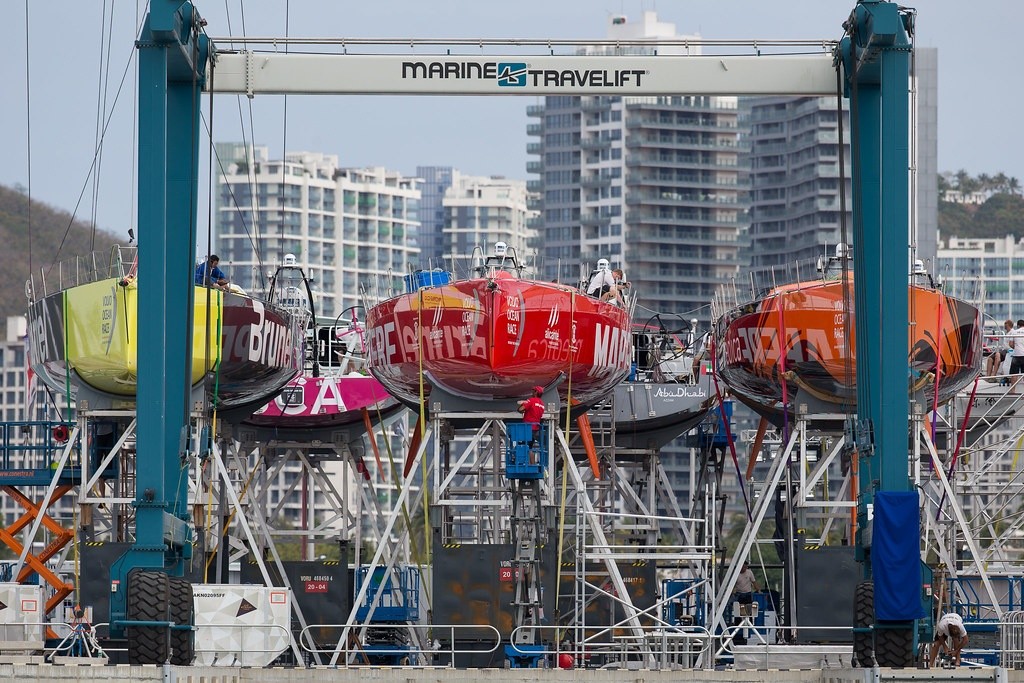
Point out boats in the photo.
[360,238,638,428]
[23,243,311,410]
[710,238,986,428]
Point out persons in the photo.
[734,560,760,626]
[587,268,631,312]
[195,254,239,293]
[930,613,968,667]
[517,385,545,465]
[982,319,1024,394]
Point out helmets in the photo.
[559,654,575,669]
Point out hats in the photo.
[531,385,544,393]
